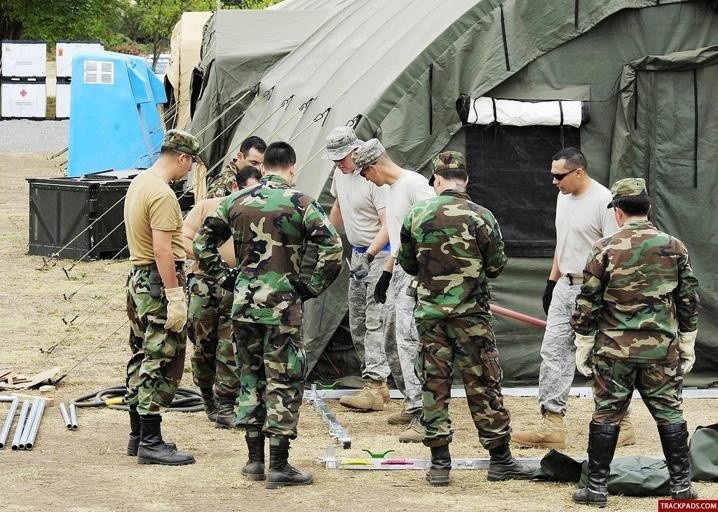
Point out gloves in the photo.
[573,332,598,378]
[375,272,393,304]
[680,329,698,373]
[542,281,558,313]
[164,286,187,334]
[348,252,375,279]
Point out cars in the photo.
[146,53,170,83]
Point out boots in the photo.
[203,389,239,430]
[660,421,697,501]
[513,412,569,450]
[426,437,453,485]
[126,406,193,464]
[242,431,266,482]
[616,415,635,450]
[340,380,391,411]
[490,443,537,482]
[572,424,622,505]
[264,434,312,490]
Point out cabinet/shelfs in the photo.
[27,175,196,263]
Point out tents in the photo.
[221,0,718,389]
[163,0,344,176]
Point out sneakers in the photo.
[402,424,423,444]
[388,409,412,423]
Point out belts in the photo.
[352,244,391,254]
[567,276,585,285]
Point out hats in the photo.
[351,138,385,179]
[429,151,468,187]
[162,128,204,164]
[605,178,648,209]
[320,127,363,160]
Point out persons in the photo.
[123,129,204,465]
[320,126,391,411]
[192,143,344,489]
[351,138,438,443]
[182,166,262,430]
[204,136,266,200]
[511,147,636,448]
[569,177,701,508]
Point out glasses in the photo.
[552,168,578,179]
